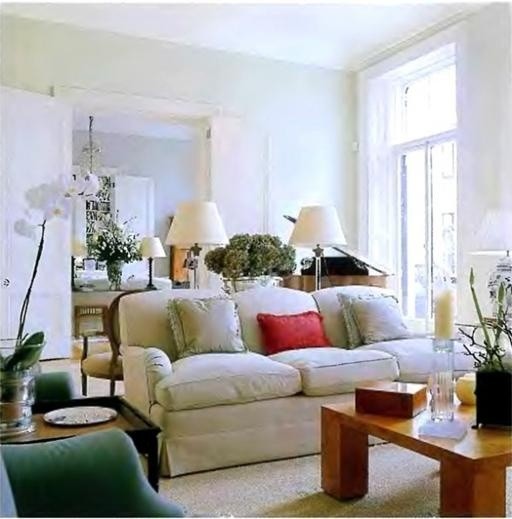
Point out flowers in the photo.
[70,209,145,289]
[0,165,101,428]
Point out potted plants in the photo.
[204,232,297,297]
[456,266,512,433]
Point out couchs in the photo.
[118,286,495,479]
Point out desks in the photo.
[73,254,128,309]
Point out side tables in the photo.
[0,393,164,494]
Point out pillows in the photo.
[164,293,249,358]
[334,291,415,350]
[256,310,334,356]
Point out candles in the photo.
[434,285,454,339]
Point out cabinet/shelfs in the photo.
[283,272,390,291]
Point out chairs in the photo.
[0,427,186,518]
[73,304,124,397]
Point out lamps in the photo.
[134,235,168,292]
[289,204,348,292]
[164,201,232,289]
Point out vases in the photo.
[106,259,123,291]
[0,331,49,438]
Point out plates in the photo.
[43,405,118,427]
